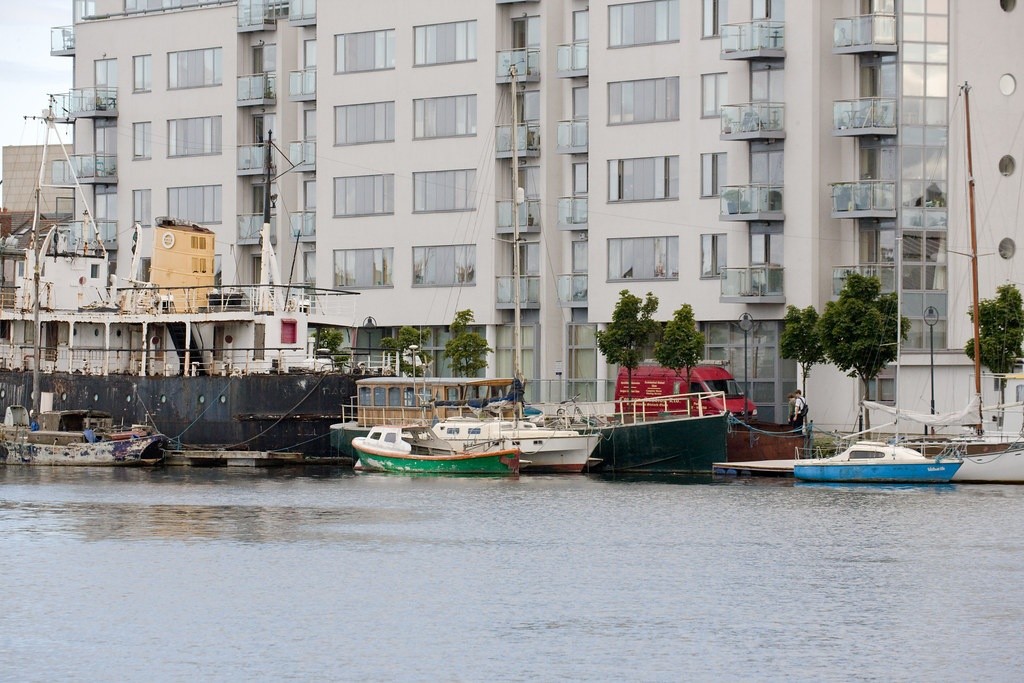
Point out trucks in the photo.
[616,364,759,421]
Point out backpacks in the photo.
[798,397,809,416]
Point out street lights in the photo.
[362,316,377,352]
[925,306,939,432]
[738,312,754,421]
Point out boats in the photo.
[793,80,1024,483]
[351,426,520,480]
[0,407,168,466]
[728,421,810,462]
[353,416,603,473]
[0,94,402,454]
[329,65,730,478]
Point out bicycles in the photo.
[556,393,586,428]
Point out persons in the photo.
[787,390,808,435]
